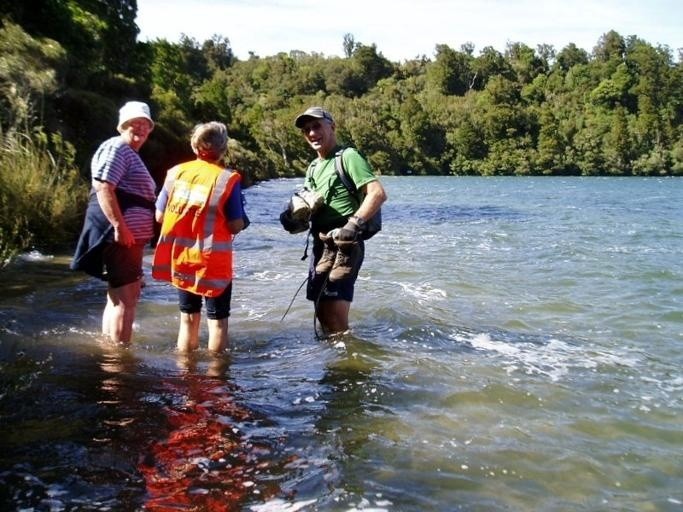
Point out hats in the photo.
[295,107,333,128]
[116,101,155,134]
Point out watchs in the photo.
[349,215,369,230]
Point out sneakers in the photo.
[297,187,324,218]
[289,194,308,221]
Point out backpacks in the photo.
[309,145,382,240]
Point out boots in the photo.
[330,227,361,281]
[315,229,338,274]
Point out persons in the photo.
[295,107,386,333]
[177,354,227,378]
[153,121,245,353]
[88,101,155,344]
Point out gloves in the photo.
[326,215,366,241]
